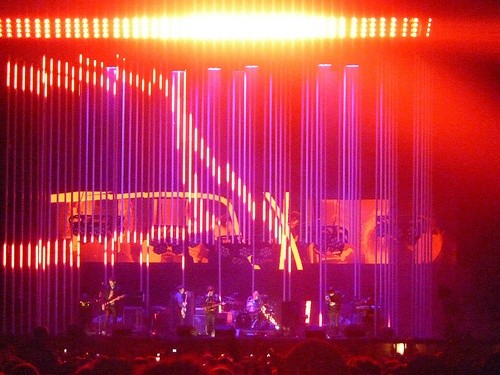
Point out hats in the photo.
[206,286,215,292]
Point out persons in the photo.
[0,340,500,375]
[175,286,189,328]
[216,295,231,312]
[204,286,219,336]
[101,277,125,336]
[249,290,263,332]
[355,291,387,329]
[326,287,342,337]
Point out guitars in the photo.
[101,296,126,310]
[180,290,188,318]
[202,301,228,313]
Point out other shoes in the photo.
[102,331,106,335]
[212,331,216,337]
